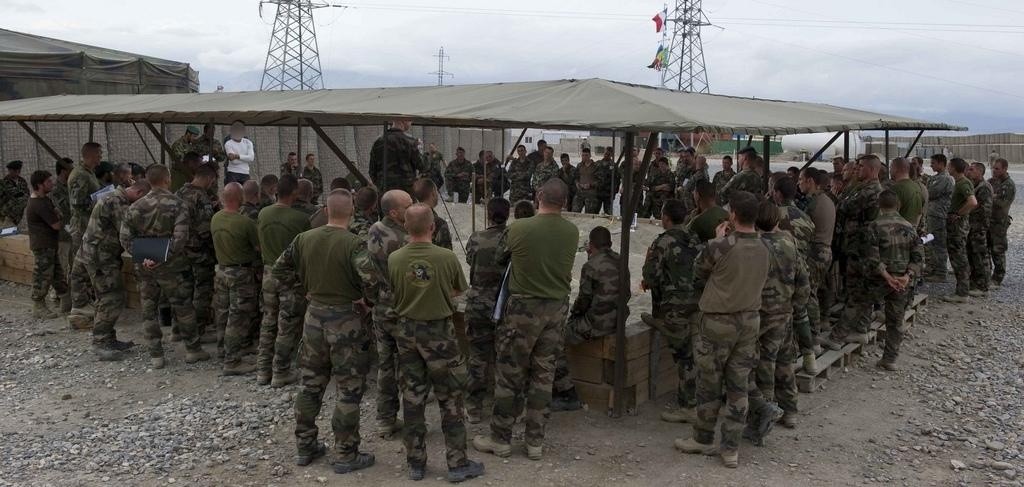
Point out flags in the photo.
[644,7,669,69]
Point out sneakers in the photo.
[151,354,166,369]
[675,436,718,457]
[777,413,799,429]
[720,444,740,469]
[925,274,947,284]
[719,401,784,448]
[942,280,1002,303]
[295,389,583,484]
[877,357,898,372]
[661,404,698,423]
[32,296,135,354]
[171,321,301,388]
[802,308,869,376]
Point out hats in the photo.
[7,160,24,170]
[187,126,201,136]
